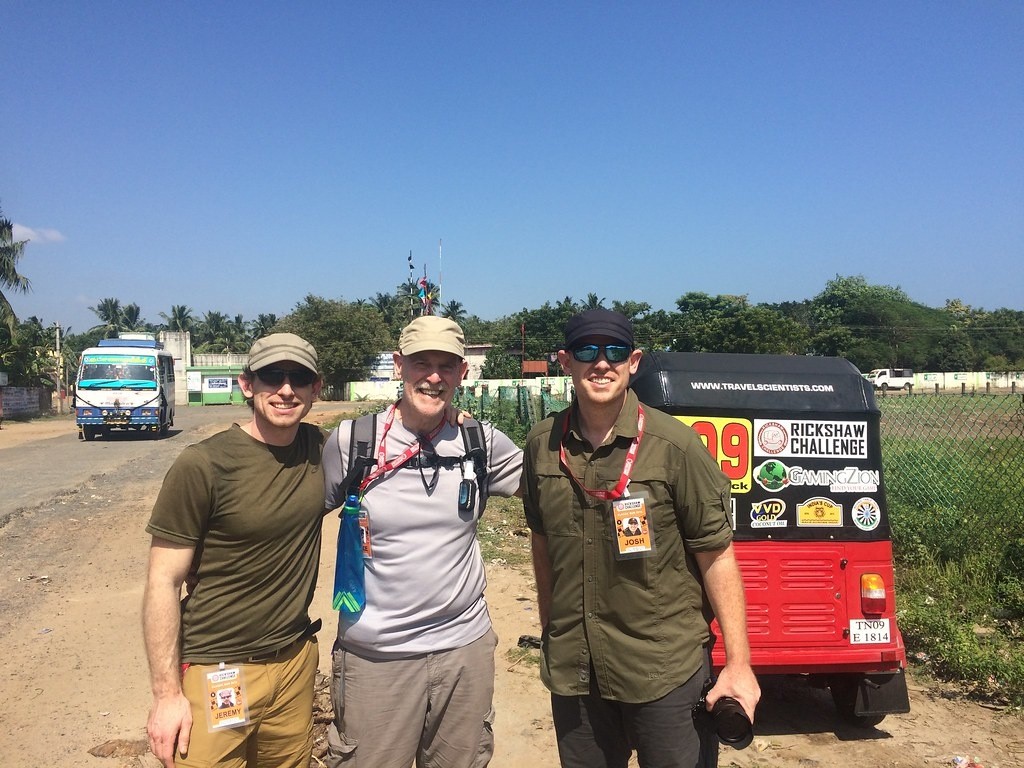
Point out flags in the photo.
[408,257,414,269]
[418,278,431,307]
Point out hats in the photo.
[397,316,465,360]
[245,332,319,375]
[565,309,635,351]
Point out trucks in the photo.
[866,367,915,391]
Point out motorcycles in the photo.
[625,355,913,728]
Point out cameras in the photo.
[690,676,766,751]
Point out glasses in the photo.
[418,432,440,489]
[567,343,632,363]
[248,368,315,388]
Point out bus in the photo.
[72,338,177,442]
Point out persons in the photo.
[523,310,761,768]
[142,332,471,768]
[218,690,234,709]
[624,518,641,536]
[322,315,522,768]
